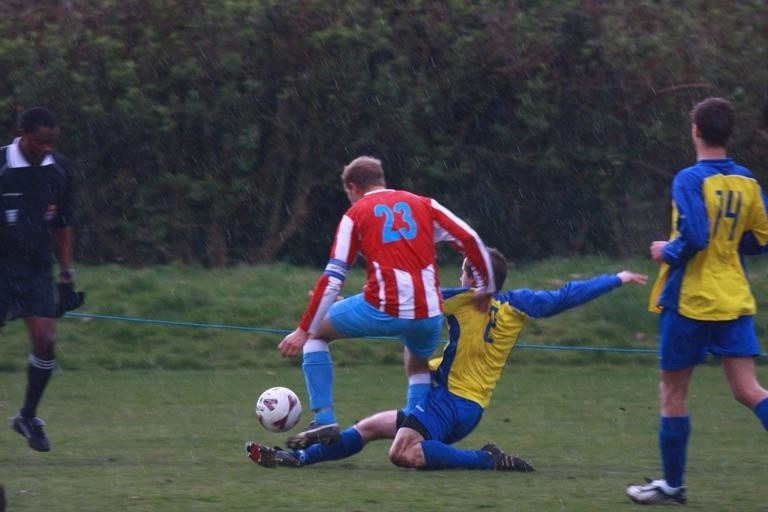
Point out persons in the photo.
[0,106,86,452]
[626,96,768,508]
[246,246,649,472]
[278,156,497,450]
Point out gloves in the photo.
[57,280,85,312]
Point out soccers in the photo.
[256,386,302,435]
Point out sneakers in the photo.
[625,478,687,505]
[481,443,536,472]
[9,408,50,452]
[245,440,301,468]
[285,411,340,450]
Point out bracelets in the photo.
[57,269,73,279]
